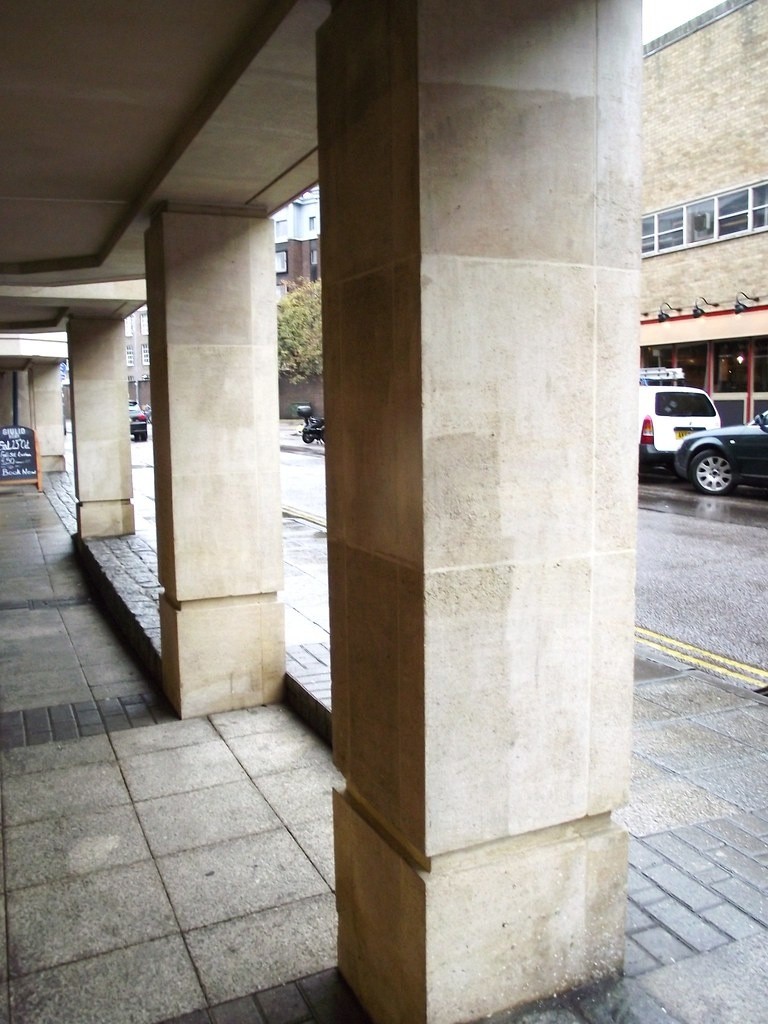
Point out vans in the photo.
[638,385,722,478]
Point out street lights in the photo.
[136,375,147,401]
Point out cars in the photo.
[675,409,768,495]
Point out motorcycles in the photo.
[297,406,326,444]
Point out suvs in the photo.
[129,401,149,440]
